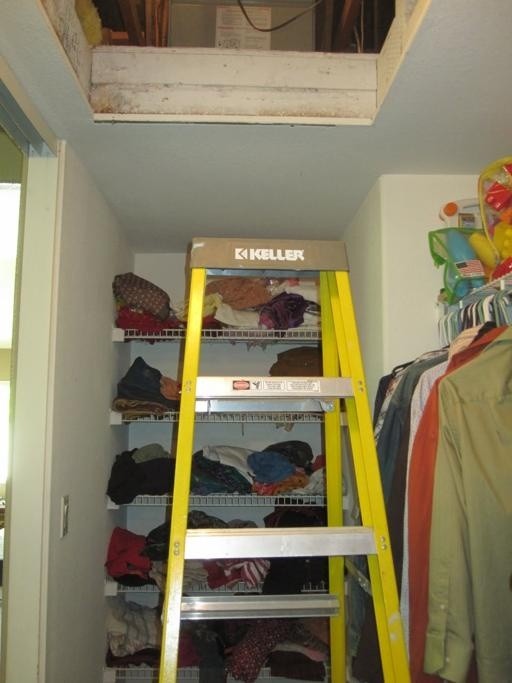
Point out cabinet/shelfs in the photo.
[105,328,351,682]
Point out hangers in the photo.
[391,272,512,390]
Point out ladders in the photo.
[160,236,410,682]
